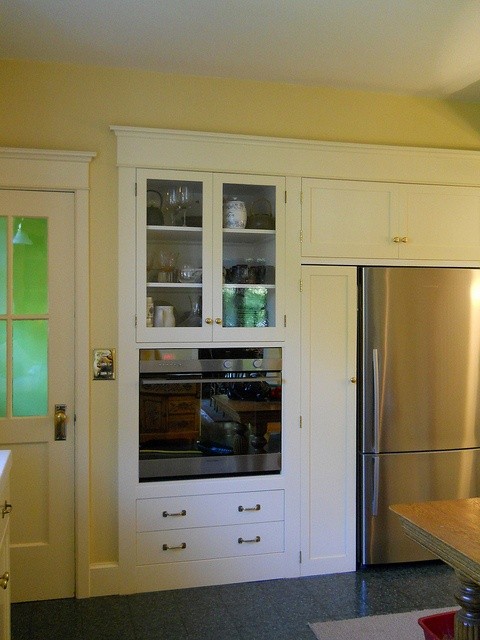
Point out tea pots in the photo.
[147,189,164,225]
[248,197,273,229]
[226,264,246,283]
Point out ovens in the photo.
[139,349,281,484]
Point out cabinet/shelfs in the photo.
[136,169,287,342]
[300,176,480,260]
[133,490,286,564]
[0,470,12,640]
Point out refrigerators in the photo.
[361,265,479,561]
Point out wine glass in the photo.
[177,183,194,226]
[166,185,175,225]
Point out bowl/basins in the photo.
[417,610,454,640]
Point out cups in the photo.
[154,305,175,327]
[247,259,266,285]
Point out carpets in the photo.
[307,606,463,640]
